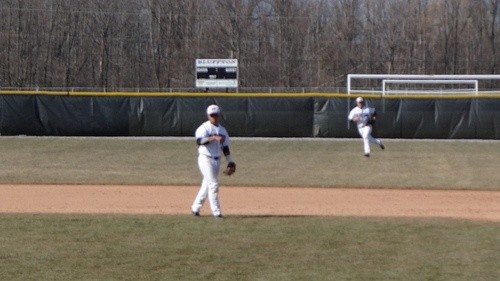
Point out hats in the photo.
[355,96,364,103]
[206,105,220,115]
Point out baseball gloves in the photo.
[223,161,236,176]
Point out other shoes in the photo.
[191,210,200,216]
[216,214,225,219]
[363,154,370,158]
[380,139,385,149]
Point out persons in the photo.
[191,105,236,218]
[348,97,385,157]
